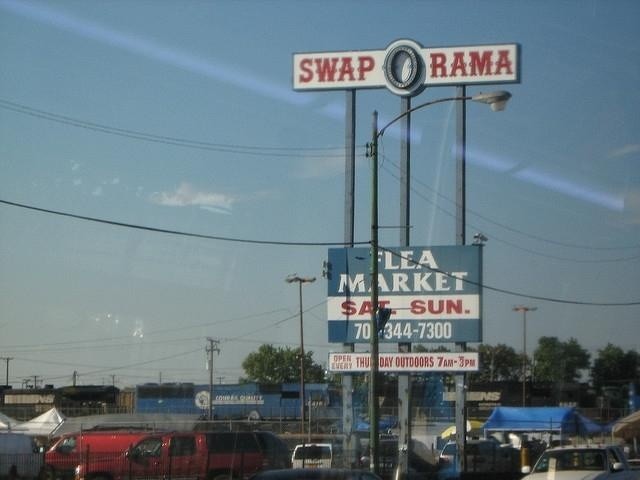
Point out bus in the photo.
[136,381,344,420]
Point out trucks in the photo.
[45,432,140,479]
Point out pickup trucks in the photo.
[520,443,640,480]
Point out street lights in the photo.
[515,306,535,406]
[370,92,510,467]
[287,276,317,433]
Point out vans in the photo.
[292,443,343,468]
[75,431,261,479]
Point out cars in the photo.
[436,436,503,477]
